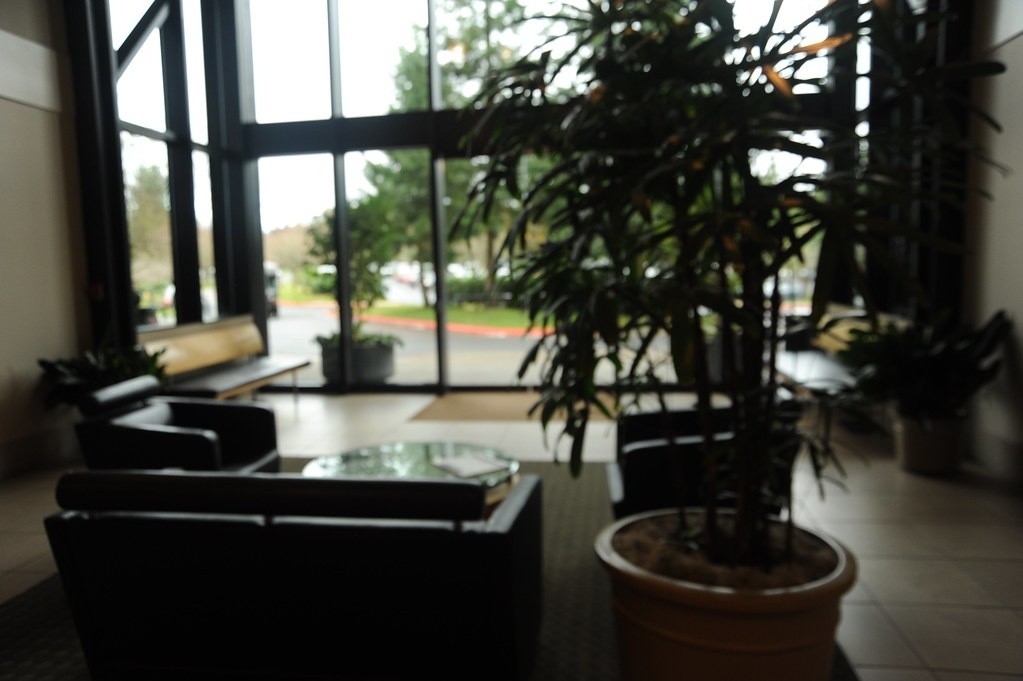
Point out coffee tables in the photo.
[302,441,520,506]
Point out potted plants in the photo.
[832,305,1013,480]
[448,1,1012,681]
[301,193,406,386]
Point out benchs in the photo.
[774,303,933,446]
[135,311,310,408]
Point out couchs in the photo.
[75,376,283,478]
[609,400,800,527]
[44,471,547,681]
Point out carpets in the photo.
[411,390,621,422]
[1,459,865,681]
